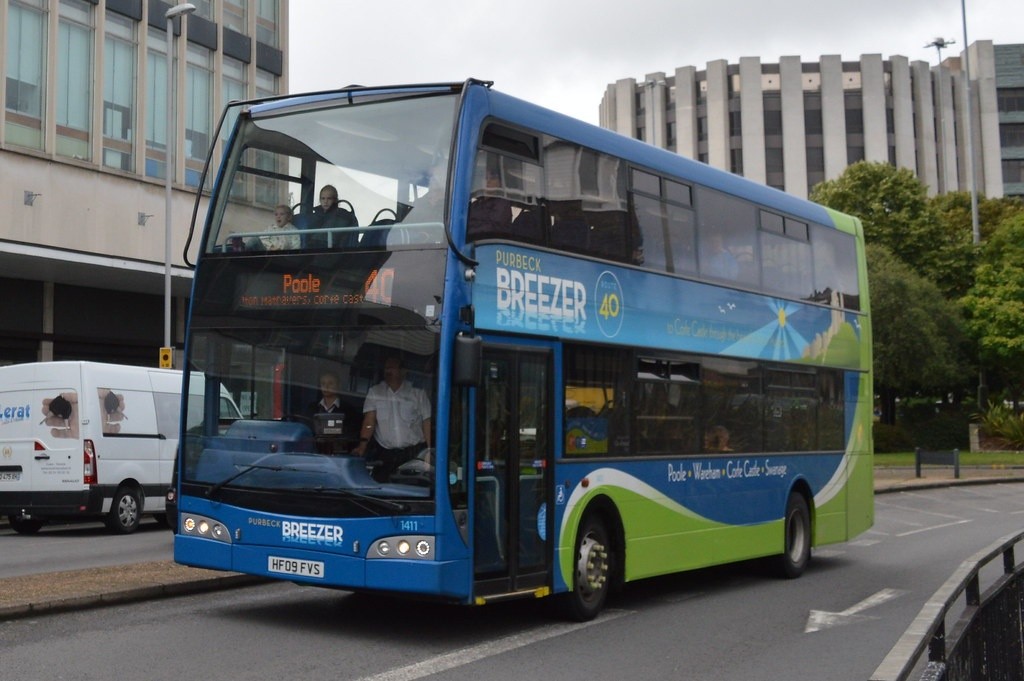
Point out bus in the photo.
[175,77,877,623]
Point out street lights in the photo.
[922,36,956,64]
[158,4,199,368]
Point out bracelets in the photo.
[358,437,368,445]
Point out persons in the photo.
[230,206,300,255]
[350,354,434,465]
[711,424,733,452]
[462,170,511,238]
[303,371,354,440]
[305,185,351,252]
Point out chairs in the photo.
[286,201,314,247]
[360,207,404,247]
[551,208,591,250]
[335,197,360,246]
[512,206,551,240]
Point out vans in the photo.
[0,359,247,534]
